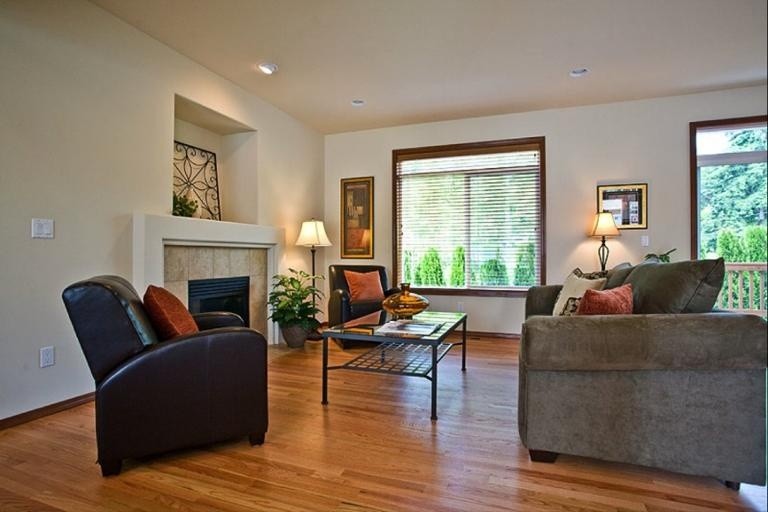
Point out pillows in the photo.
[552,272,607,316]
[603,262,636,290]
[622,257,725,314]
[143,284,200,341]
[344,270,385,305]
[575,283,633,315]
[573,268,608,280]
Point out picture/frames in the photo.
[596,183,648,229]
[340,176,374,260]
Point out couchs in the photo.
[327,264,402,350]
[62,275,269,477]
[518,257,768,491]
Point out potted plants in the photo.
[265,268,325,348]
[171,192,198,217]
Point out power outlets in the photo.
[40,346,55,368]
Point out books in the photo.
[374,318,448,336]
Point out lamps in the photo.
[570,67,589,77]
[351,99,365,107]
[587,213,622,271]
[294,218,334,341]
[257,61,279,75]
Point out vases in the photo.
[183,187,202,218]
